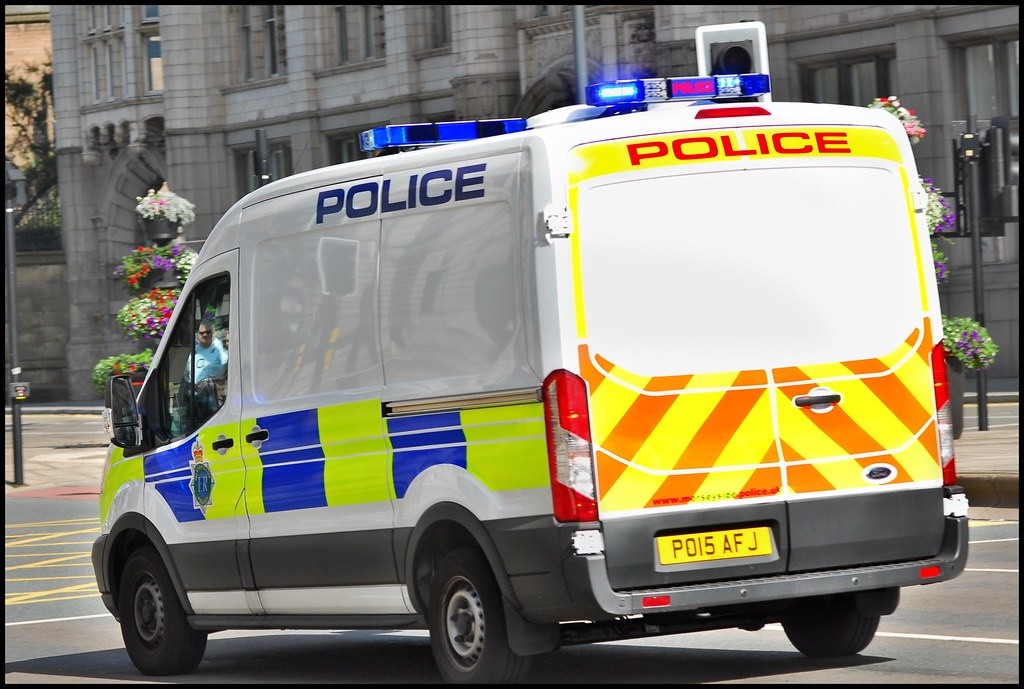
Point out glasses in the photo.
[199,330,212,336]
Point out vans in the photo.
[89,73,974,689]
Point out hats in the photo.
[222,334,228,341]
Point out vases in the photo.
[143,268,180,288]
[946,356,966,440]
[128,369,147,382]
[144,219,178,238]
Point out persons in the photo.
[178,320,228,442]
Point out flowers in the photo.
[919,178,957,234]
[941,314,1000,371]
[194,304,226,344]
[116,288,182,344]
[113,246,199,289]
[135,189,195,225]
[931,243,951,284]
[93,349,154,392]
[867,95,926,146]
